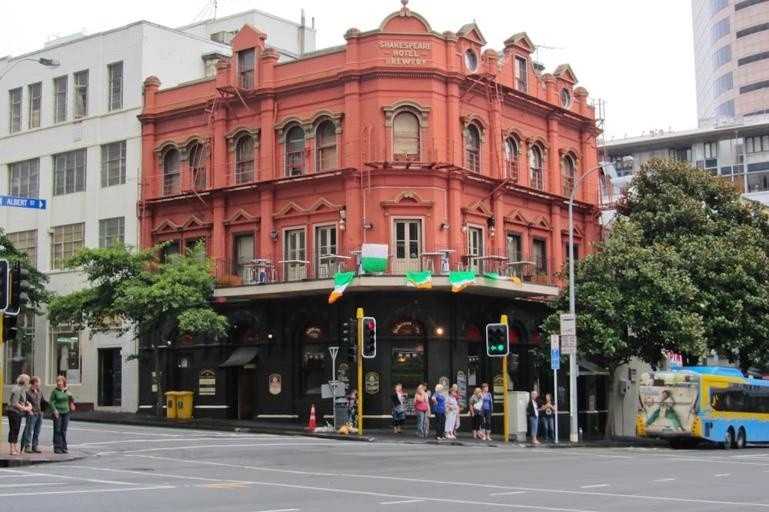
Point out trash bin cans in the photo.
[164,390,194,420]
[334,397,349,431]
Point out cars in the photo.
[391,359,425,387]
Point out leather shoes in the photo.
[8,447,71,458]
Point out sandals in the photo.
[390,429,559,445]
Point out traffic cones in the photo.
[304,403,318,431]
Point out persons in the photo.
[59,345,69,379]
[25,376,44,453]
[49,375,74,454]
[6,373,33,455]
[346,388,358,428]
[645,390,686,433]
[391,379,556,444]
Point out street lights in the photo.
[565,152,637,444]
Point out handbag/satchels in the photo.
[415,398,428,412]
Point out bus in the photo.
[634,363,769,451]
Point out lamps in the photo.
[272,230,278,241]
[488,226,496,241]
[461,222,468,233]
[361,223,373,229]
[339,218,345,232]
[441,223,449,229]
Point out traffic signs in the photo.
[0,196,49,210]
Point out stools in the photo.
[242,253,353,286]
[418,249,538,282]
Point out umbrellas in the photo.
[20,425,26,452]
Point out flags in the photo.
[327,271,355,305]
[449,270,475,293]
[482,270,522,285]
[406,270,432,289]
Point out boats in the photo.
[10,260,32,309]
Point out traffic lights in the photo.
[0,258,9,312]
[484,322,510,359]
[341,318,358,347]
[361,316,377,359]
[0,312,17,343]
